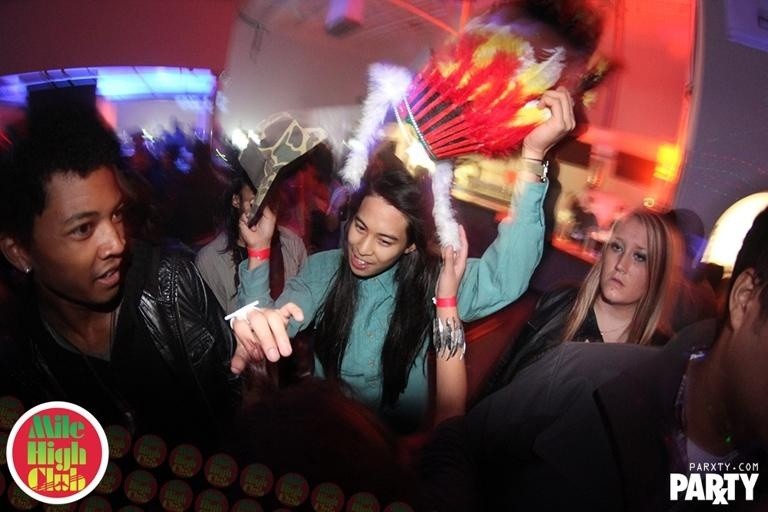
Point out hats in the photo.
[238,111,328,225]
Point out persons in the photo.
[0,85,768,512]
